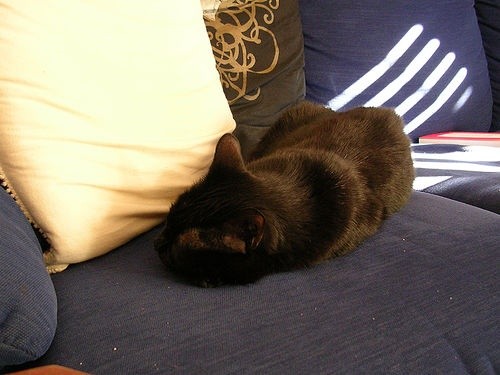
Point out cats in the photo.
[152,102,416,290]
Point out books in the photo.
[418,131,500,147]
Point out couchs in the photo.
[0,144,500,375]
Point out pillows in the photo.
[0,0,237,271]
[201,0,306,154]
[300,0,492,144]
